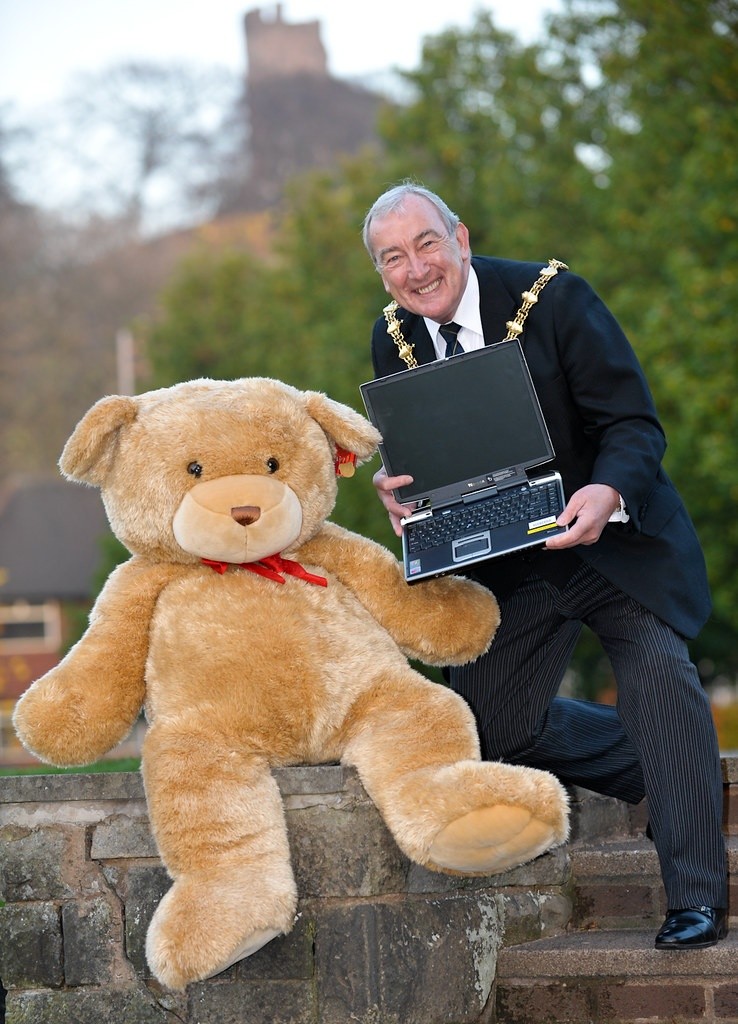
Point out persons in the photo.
[363,185,731,949]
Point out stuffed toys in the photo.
[12,375,572,988]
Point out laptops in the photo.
[360,337,570,584]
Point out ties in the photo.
[439,322,465,359]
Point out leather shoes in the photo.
[655,904,728,949]
